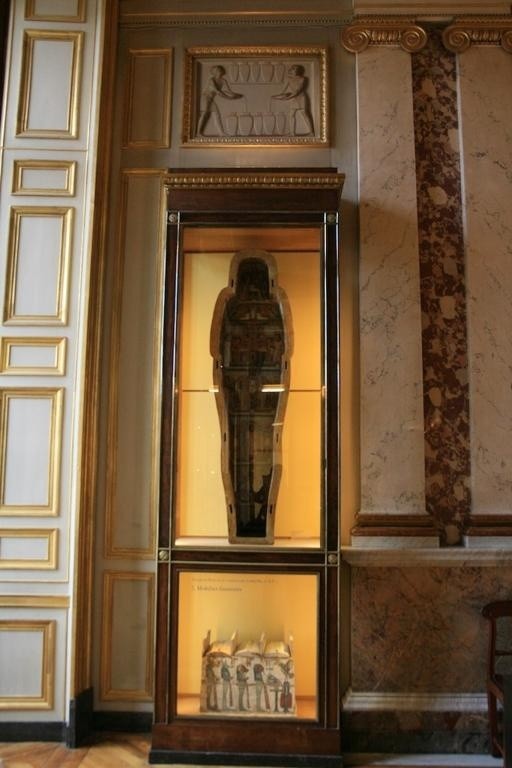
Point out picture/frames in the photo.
[180,45,332,151]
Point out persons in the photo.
[197,65,242,139]
[271,64,315,138]
